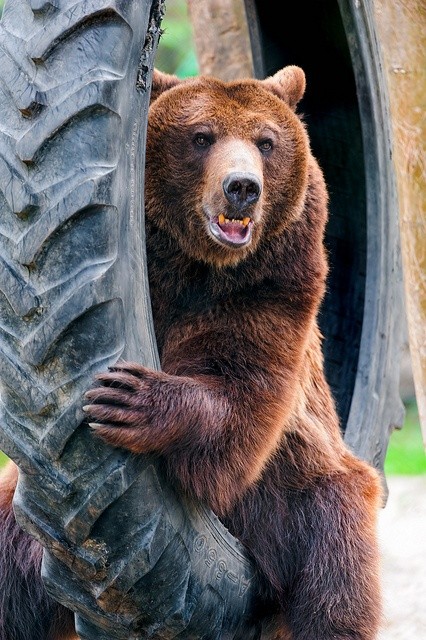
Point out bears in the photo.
[0,65,382,640]
